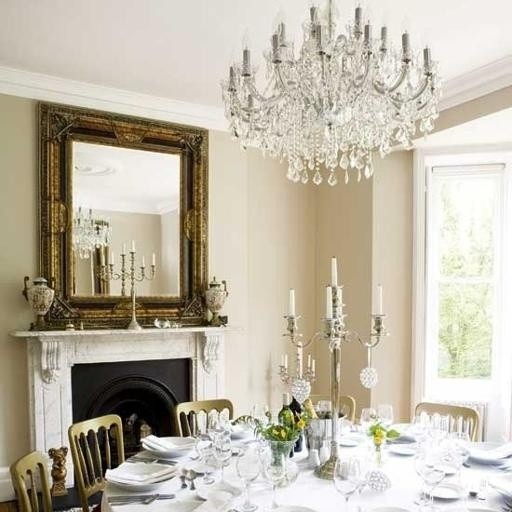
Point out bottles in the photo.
[279,392,303,457]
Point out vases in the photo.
[372,441,386,469]
[264,440,299,487]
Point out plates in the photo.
[470,438,512,505]
[107,436,195,493]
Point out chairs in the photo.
[409,401,483,442]
[8,450,53,511]
[172,399,233,436]
[67,413,126,511]
[293,394,357,425]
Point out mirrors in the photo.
[35,99,210,322]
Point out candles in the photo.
[99,243,146,270]
[280,252,391,378]
[103,238,157,276]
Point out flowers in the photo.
[365,416,401,455]
[231,406,315,464]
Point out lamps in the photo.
[219,0,441,189]
[67,204,112,259]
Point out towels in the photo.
[105,468,180,484]
[139,432,211,452]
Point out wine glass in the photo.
[198,401,471,511]
